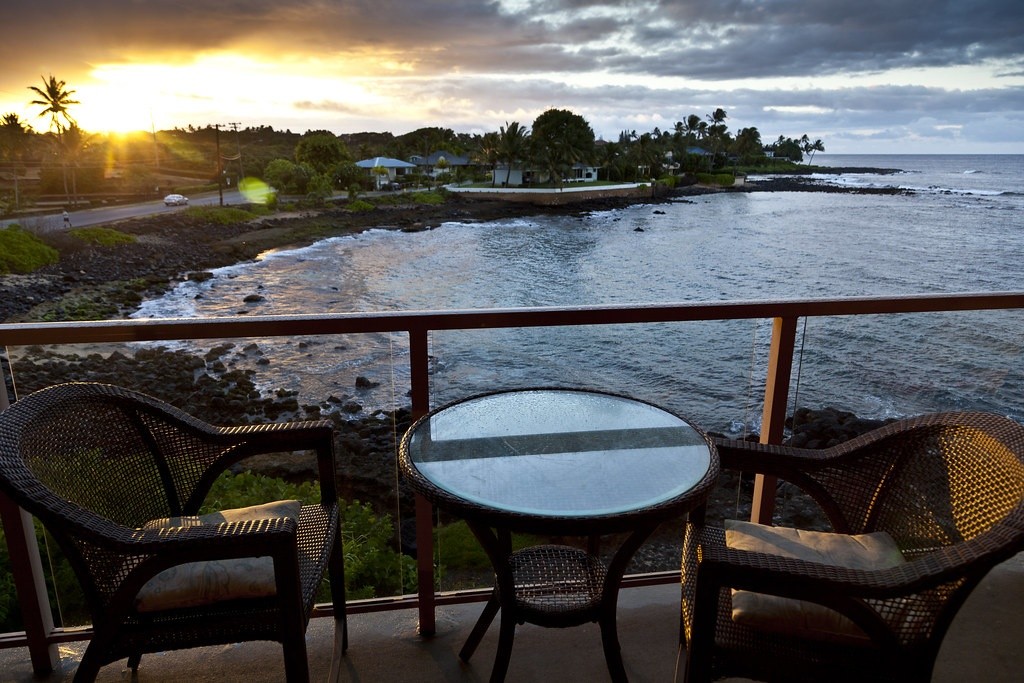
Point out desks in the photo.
[399,384,720,683]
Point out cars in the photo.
[163,193,189,206]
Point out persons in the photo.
[62,209,72,228]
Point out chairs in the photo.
[0,382,347,683]
[676,412,1024,683]
